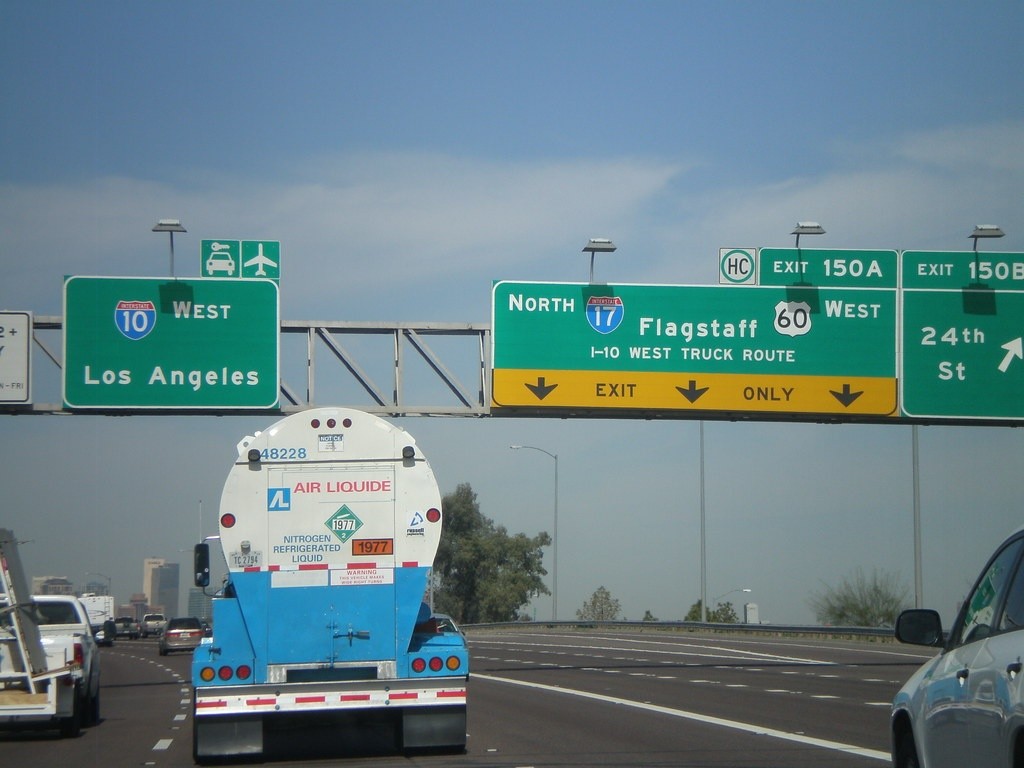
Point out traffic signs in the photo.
[482,248,900,424]
[899,251,1024,422]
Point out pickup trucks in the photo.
[0,597,116,739]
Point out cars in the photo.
[882,530,1023,768]
[77,589,213,656]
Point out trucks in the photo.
[182,409,472,766]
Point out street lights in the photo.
[712,588,751,621]
[510,444,559,622]
